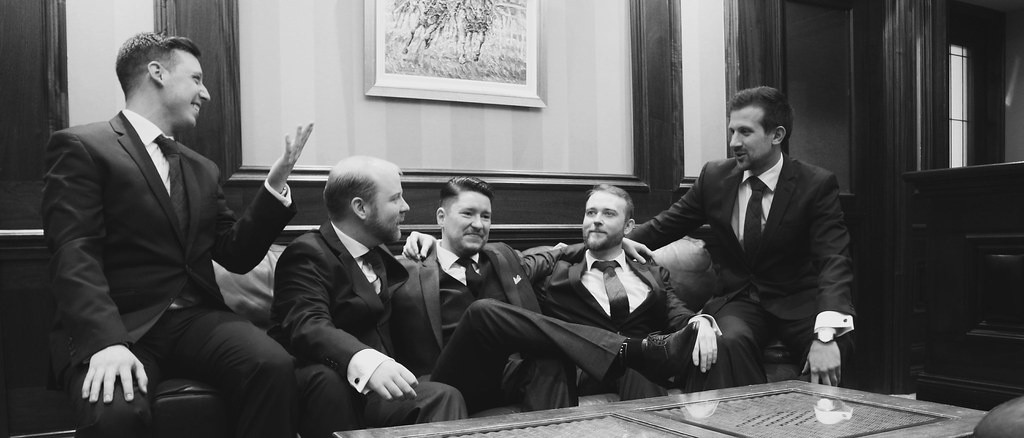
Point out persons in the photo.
[552,86,856,387]
[403,183,722,400]
[394,174,700,412]
[40,33,313,438]
[266,158,468,438]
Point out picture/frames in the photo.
[363,0,549,109]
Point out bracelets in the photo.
[280,186,287,196]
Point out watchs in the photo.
[813,329,838,343]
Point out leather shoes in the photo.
[635,322,698,389]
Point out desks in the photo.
[332,378,987,438]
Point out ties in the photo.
[592,260,630,327]
[455,257,481,299]
[745,175,766,267]
[361,249,390,306]
[154,134,189,242]
